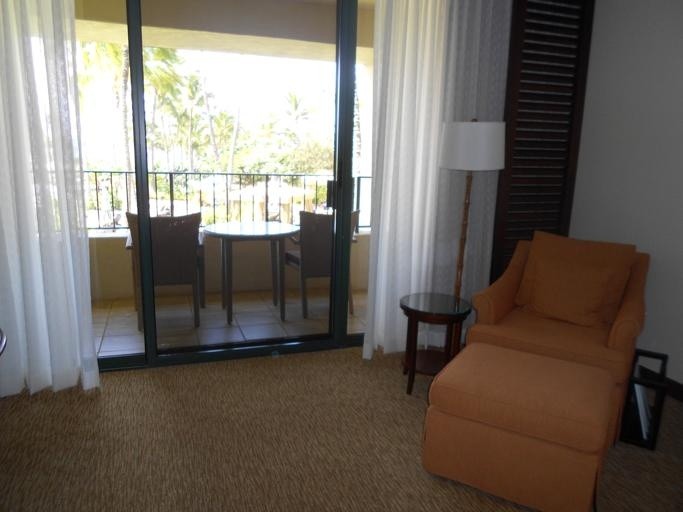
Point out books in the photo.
[632,363,653,439]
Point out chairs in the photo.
[424,239,650,511]
[125,210,205,331]
[284,209,360,319]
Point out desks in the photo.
[204,220,300,324]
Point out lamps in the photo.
[440,118,505,299]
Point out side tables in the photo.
[400,292,471,395]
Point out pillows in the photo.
[514,229,637,329]
